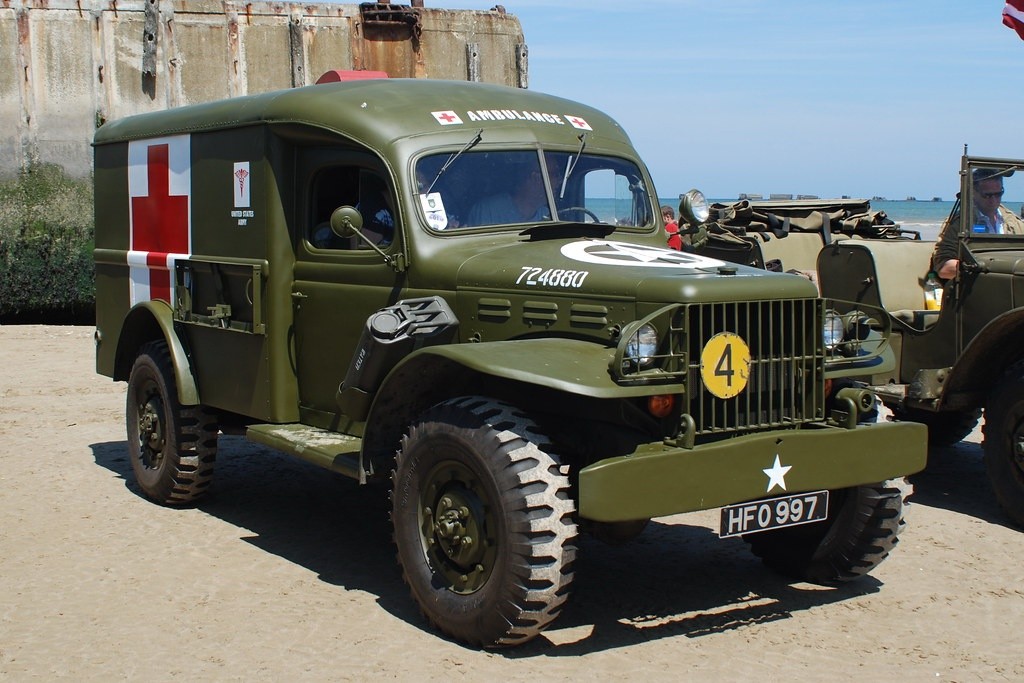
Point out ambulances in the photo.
[90,70,928,651]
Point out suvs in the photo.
[677,143,1024,527]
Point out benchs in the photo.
[744,230,865,273]
[830,239,952,331]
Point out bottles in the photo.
[925,272,943,311]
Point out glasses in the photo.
[984,188,1010,199]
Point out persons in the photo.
[463,146,564,230]
[931,166,1024,281]
[660,205,682,253]
[312,154,480,259]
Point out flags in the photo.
[1002,1,1024,40]
